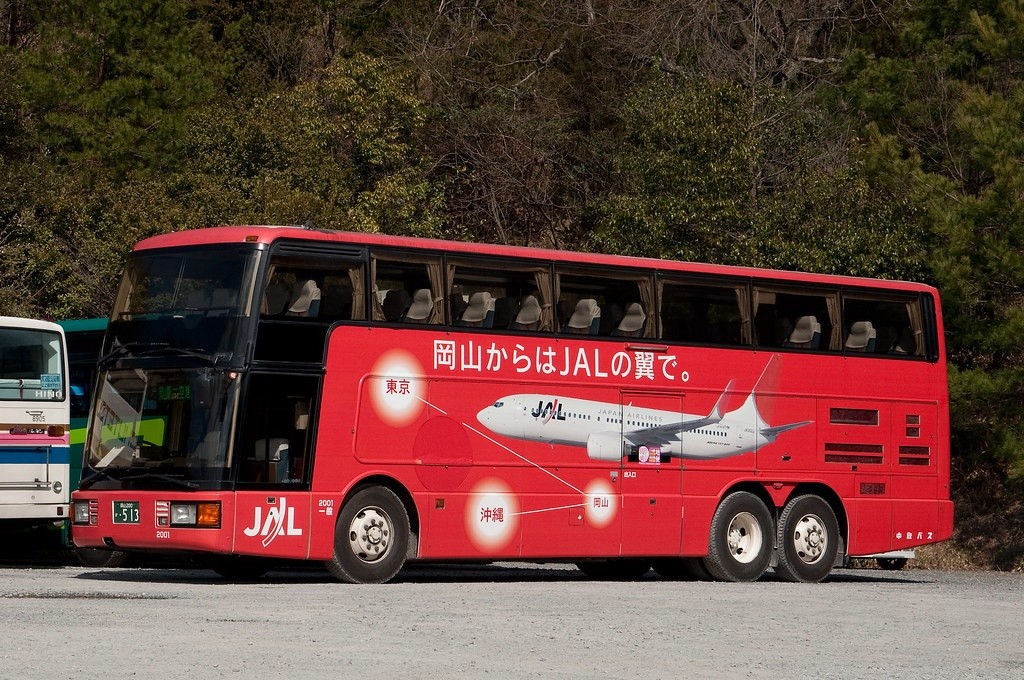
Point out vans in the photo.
[0,316,72,521]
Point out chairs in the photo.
[840,320,878,353]
[374,287,434,323]
[783,314,822,350]
[553,293,650,342]
[182,279,352,321]
[448,291,541,333]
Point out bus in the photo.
[66,223,952,588]
[57,309,186,567]
